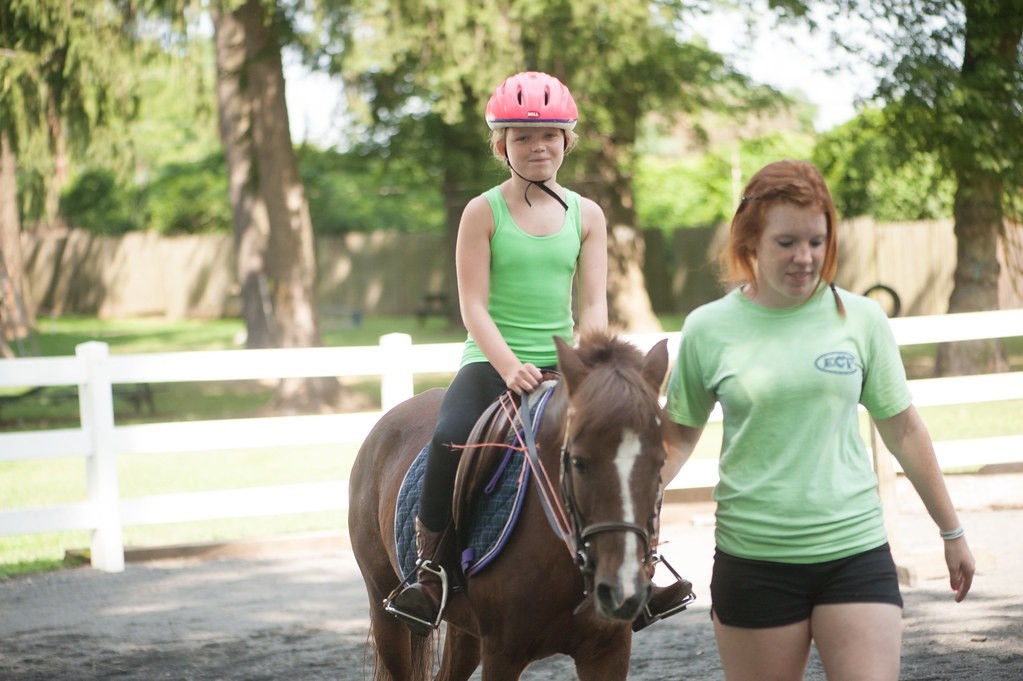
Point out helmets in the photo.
[485,71,578,130]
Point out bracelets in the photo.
[937,525,966,542]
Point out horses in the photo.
[347,333,669,681]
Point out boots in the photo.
[394,515,453,637]
[632,579,692,632]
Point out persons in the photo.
[380,72,694,639]
[657,158,976,681]
